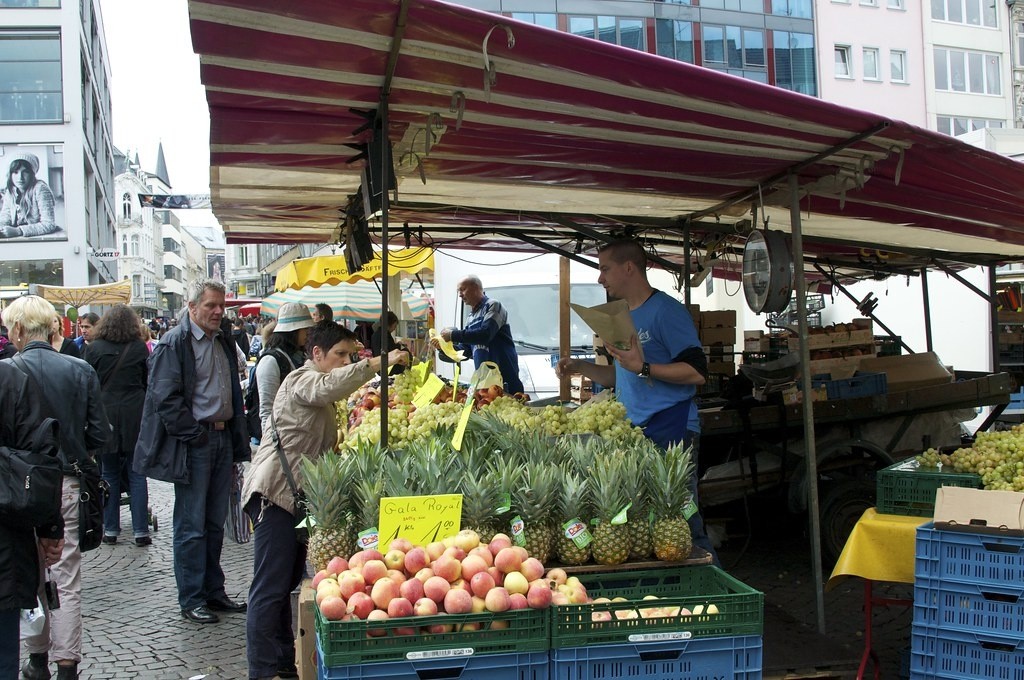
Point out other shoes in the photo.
[103,536,117,544]
[22,658,50,680]
[58,673,79,680]
[136,537,152,547]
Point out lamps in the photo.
[74,246,80,254]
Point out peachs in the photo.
[346,385,530,430]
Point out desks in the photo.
[825,506,933,680]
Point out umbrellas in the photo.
[260,279,428,320]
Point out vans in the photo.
[434,246,699,404]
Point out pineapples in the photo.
[299,414,693,577]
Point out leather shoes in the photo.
[182,606,218,623]
[207,595,247,612]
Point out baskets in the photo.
[313,589,549,666]
[875,454,1024,680]
[550,635,763,680]
[549,564,765,648]
[311,632,550,680]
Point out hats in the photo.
[273,303,314,332]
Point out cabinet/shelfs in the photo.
[988,282,1024,433]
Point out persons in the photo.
[430,276,524,398]
[0,295,112,680]
[132,278,247,625]
[0,304,413,547]
[0,153,55,238]
[555,240,720,565]
[240,319,410,680]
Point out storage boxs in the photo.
[296,564,764,680]
[899,522,1024,680]
[875,454,980,518]
[679,294,952,405]
[551,353,609,407]
[1002,386,1024,409]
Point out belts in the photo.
[200,421,231,431]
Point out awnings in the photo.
[273,248,436,311]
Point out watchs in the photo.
[637,362,650,378]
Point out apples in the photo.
[312,530,718,639]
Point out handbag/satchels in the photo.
[293,496,309,556]
[0,417,63,520]
[244,348,292,439]
[224,462,249,543]
[79,473,103,552]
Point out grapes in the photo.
[336,400,647,455]
[915,423,1024,494]
[392,369,423,404]
